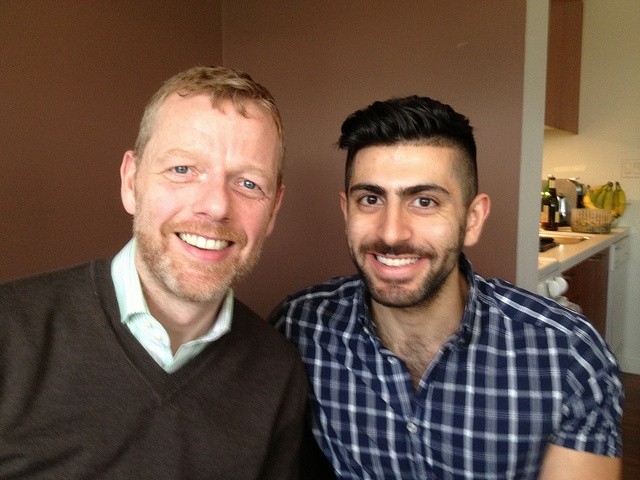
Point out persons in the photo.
[0,67,334,480]
[264,96,624,480]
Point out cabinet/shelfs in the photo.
[538,226,631,355]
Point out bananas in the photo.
[583,181,626,218]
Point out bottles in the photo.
[540,177,560,231]
